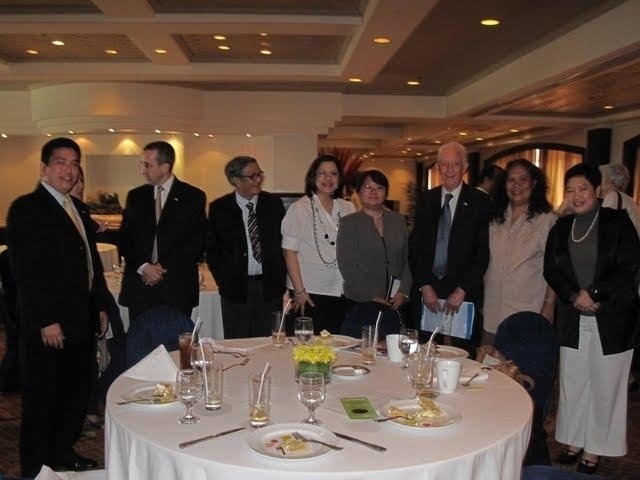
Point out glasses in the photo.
[239,171,265,182]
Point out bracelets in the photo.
[544,297,558,309]
[293,286,307,298]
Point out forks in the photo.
[291,431,343,452]
[463,372,478,387]
[222,358,249,372]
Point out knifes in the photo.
[332,430,386,454]
[179,426,246,452]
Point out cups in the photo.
[362,326,377,365]
[246,372,270,426]
[437,360,463,394]
[178,332,194,368]
[272,311,286,348]
[386,334,402,363]
[201,360,223,411]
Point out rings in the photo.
[591,304,594,307]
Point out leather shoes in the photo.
[52,455,98,472]
[557,445,600,475]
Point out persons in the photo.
[279,153,357,337]
[4,136,111,479]
[481,158,558,348]
[206,155,287,339]
[596,161,639,237]
[542,161,640,476]
[407,140,490,359]
[117,140,209,373]
[65,161,112,439]
[470,162,503,200]
[336,169,414,337]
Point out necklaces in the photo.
[571,209,600,244]
[308,194,343,268]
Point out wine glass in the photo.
[175,369,201,425]
[297,372,325,428]
[190,343,214,394]
[400,327,435,396]
[294,316,313,346]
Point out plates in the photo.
[331,364,370,378]
[377,398,464,430]
[120,380,182,407]
[246,422,341,460]
[313,334,363,350]
[432,344,469,361]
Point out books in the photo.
[419,296,476,341]
[384,274,401,305]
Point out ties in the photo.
[150,186,165,265]
[432,193,455,280]
[63,194,93,282]
[245,202,264,264]
